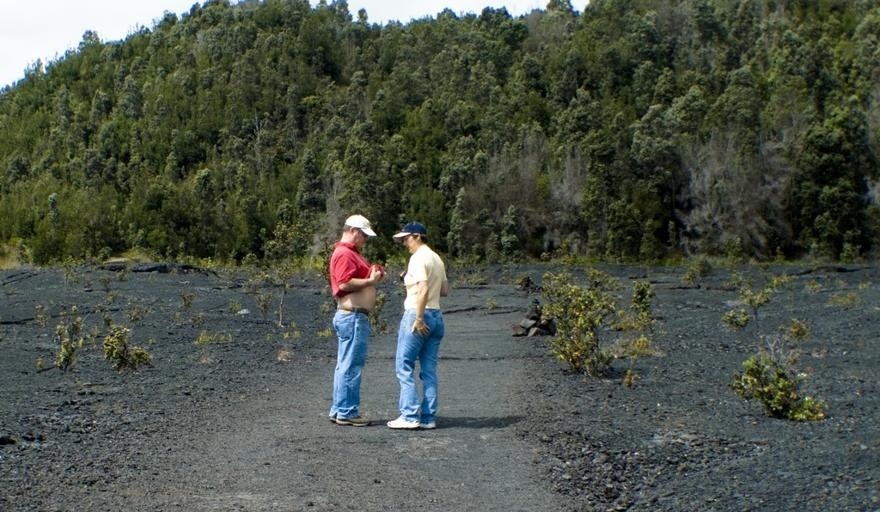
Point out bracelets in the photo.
[416,316,424,323]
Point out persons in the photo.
[386,221,450,430]
[329,213,388,428]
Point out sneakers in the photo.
[328,412,370,427]
[421,421,437,430]
[387,414,421,430]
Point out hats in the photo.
[393,222,426,241]
[345,215,377,237]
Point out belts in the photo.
[341,307,370,315]
